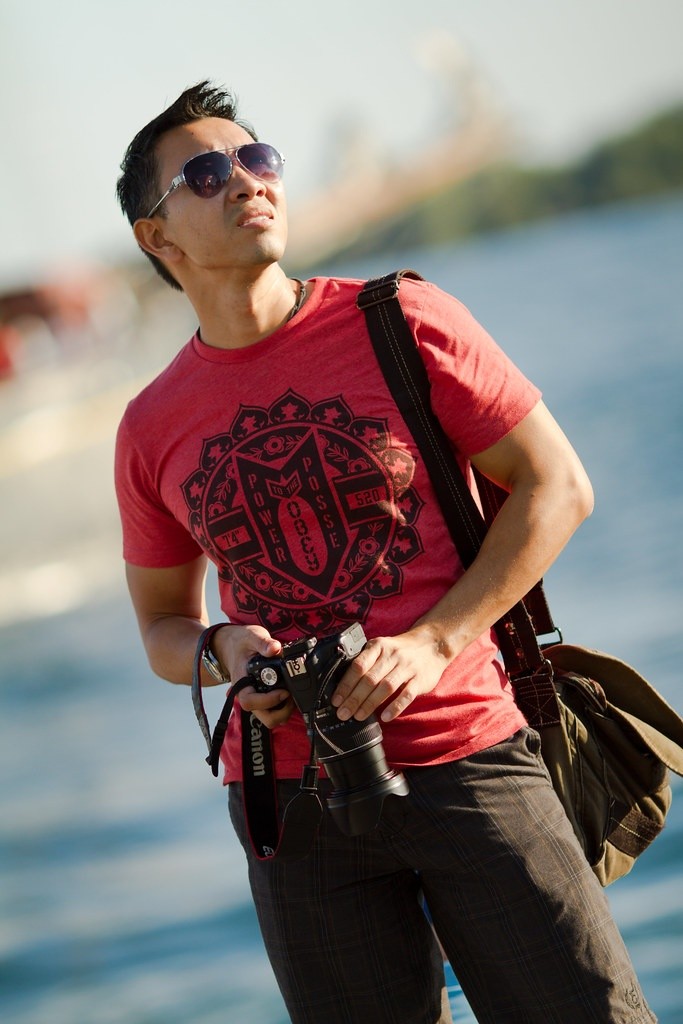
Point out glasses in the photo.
[146,142,285,219]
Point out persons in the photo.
[114,80,657,1024]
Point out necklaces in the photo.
[287,277,308,321]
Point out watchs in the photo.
[202,636,231,684]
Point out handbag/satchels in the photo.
[507,626,683,887]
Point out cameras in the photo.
[243,620,410,839]
